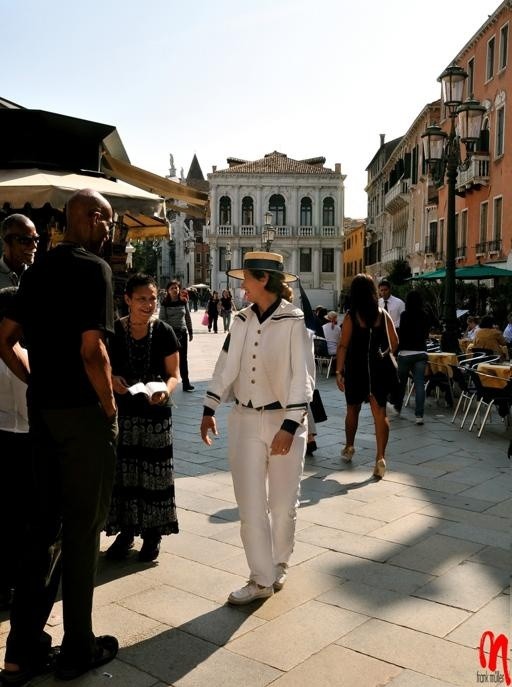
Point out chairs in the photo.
[307,318,512,457]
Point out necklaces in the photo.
[126,318,153,373]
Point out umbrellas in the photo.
[407,259,512,314]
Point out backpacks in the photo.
[201,313,209,326]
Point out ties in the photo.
[384,300,388,312]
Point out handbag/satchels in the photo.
[380,348,400,396]
[310,389,328,423]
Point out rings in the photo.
[282,448,286,452]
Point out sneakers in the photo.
[138,534,162,562]
[306,441,317,454]
[273,562,290,591]
[228,579,274,606]
[182,384,194,392]
[415,415,424,425]
[105,533,135,560]
[386,401,400,417]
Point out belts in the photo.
[236,398,283,410]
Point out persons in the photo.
[105,271,183,563]
[0,210,40,615]
[333,269,404,481]
[279,282,327,458]
[0,189,123,685]
[198,248,314,608]
[156,275,512,428]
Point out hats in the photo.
[323,311,339,322]
[226,251,300,284]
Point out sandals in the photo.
[54,635,119,681]
[0,634,61,687]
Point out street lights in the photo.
[259,210,278,252]
[224,240,233,291]
[419,60,487,396]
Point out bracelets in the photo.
[335,369,344,376]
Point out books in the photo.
[126,380,169,402]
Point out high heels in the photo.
[373,457,386,478]
[340,446,355,463]
